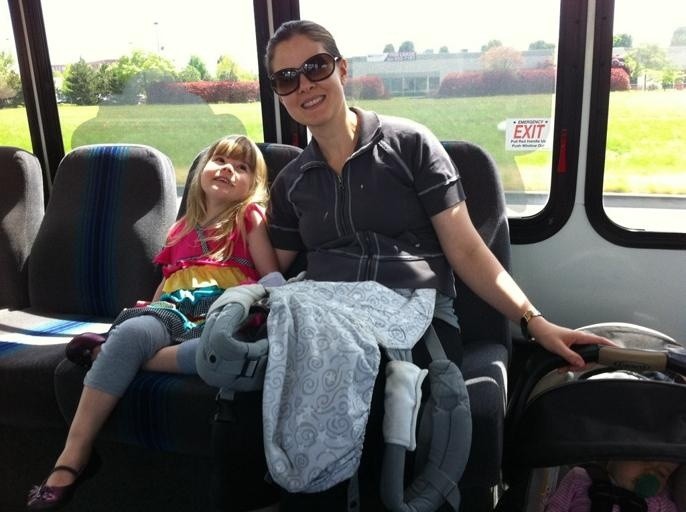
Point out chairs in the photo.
[0,140,518,510]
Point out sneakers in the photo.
[66,333,106,370]
[23,441,102,512]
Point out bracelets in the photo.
[518,309,545,345]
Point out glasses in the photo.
[271,53,338,96]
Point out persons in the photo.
[22,134,283,512]
[253,17,620,511]
[547,460,680,512]
